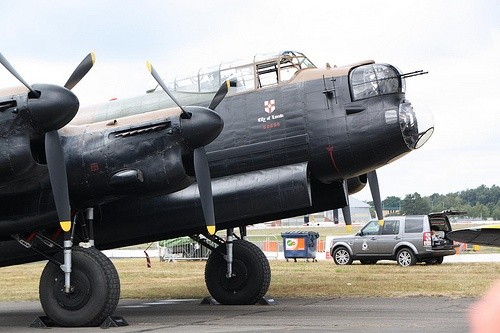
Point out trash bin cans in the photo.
[282,231,319,262]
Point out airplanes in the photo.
[0,49,434,327]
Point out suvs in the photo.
[330,210,467,267]
